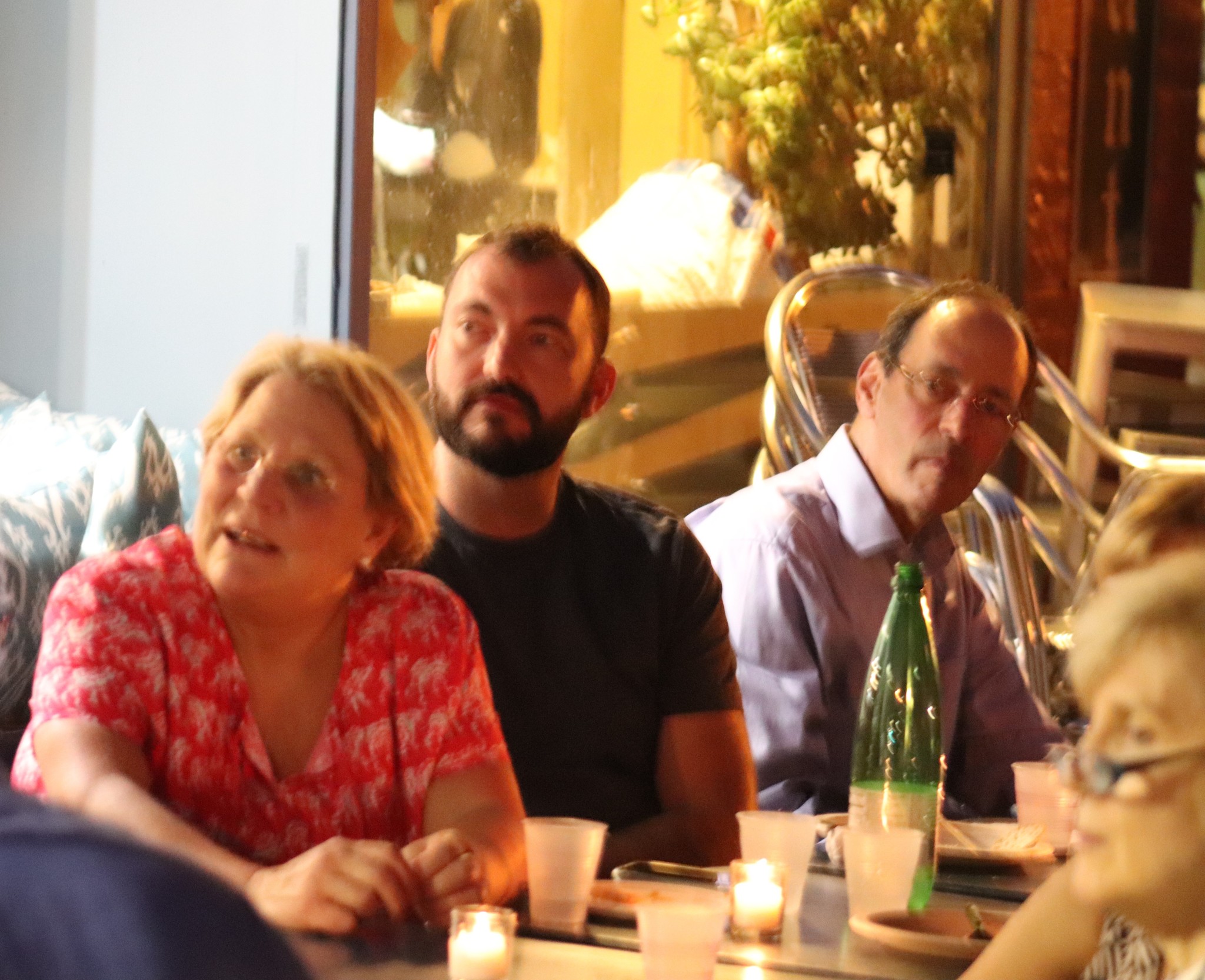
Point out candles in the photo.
[448,912,506,980]
[734,858,782,935]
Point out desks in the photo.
[338,812,1068,980]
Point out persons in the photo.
[687,277,1064,856]
[8,337,531,965]
[954,467,1205,980]
[411,220,755,878]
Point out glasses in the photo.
[1055,743,1205,798]
[887,357,1022,428]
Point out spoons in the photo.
[966,902,992,939]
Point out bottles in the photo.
[848,560,944,914]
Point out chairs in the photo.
[750,261,1156,735]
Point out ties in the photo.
[888,523,933,620]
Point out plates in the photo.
[587,878,728,920]
[849,907,1026,963]
[937,820,1062,862]
[814,813,850,834]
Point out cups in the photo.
[520,815,609,936]
[734,811,820,911]
[446,905,518,980]
[718,861,792,942]
[634,905,719,980]
[836,826,923,915]
[1012,760,1075,849]
